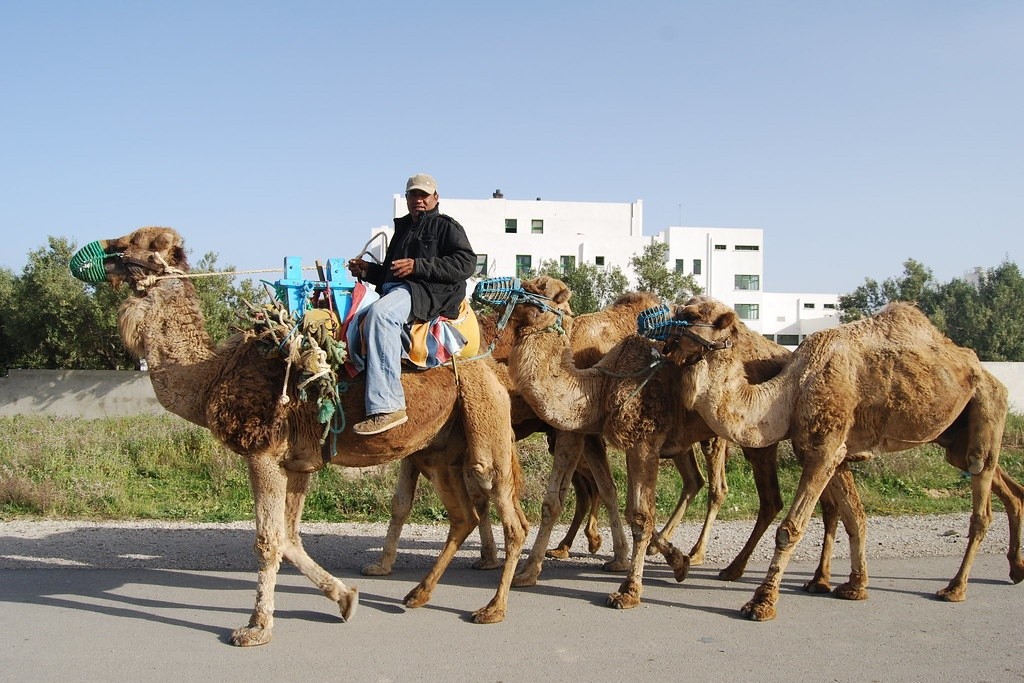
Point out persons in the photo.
[349,172,476,435]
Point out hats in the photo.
[405,174,439,195]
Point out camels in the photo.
[64,225,1024,646]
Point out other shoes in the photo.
[352,410,408,434]
